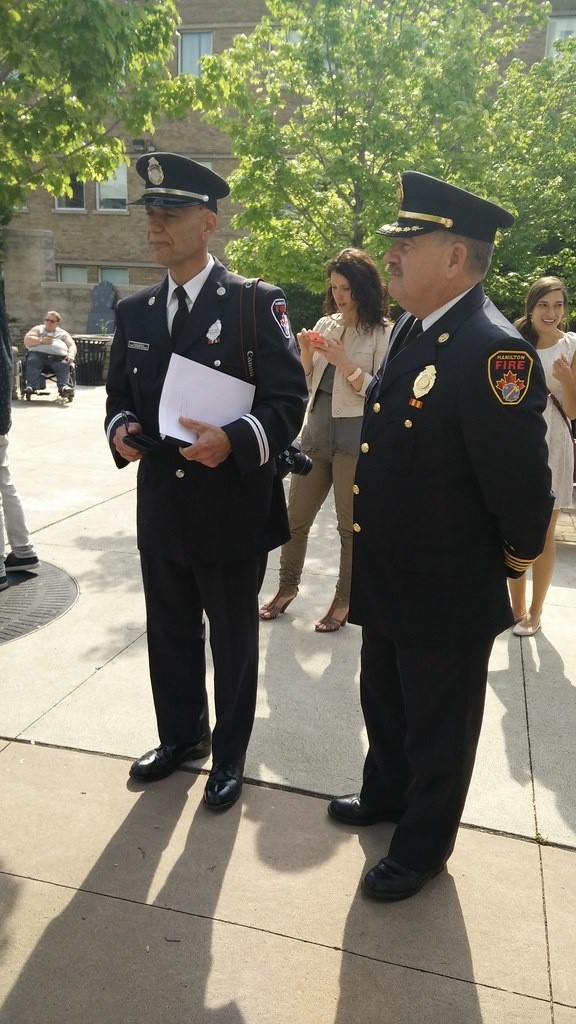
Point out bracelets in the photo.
[347,366,362,383]
[40,337,44,345]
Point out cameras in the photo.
[276,441,313,480]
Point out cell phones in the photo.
[308,331,326,349]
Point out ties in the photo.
[169,286,190,337]
[390,318,422,360]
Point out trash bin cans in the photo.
[73,338,110,386]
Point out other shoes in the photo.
[61,386,73,398]
[24,386,34,393]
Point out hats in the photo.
[124,152,230,215]
[375,169,515,244]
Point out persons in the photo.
[0,262,43,592]
[102,151,311,811]
[22,311,78,396]
[325,168,557,902]
[256,248,397,633]
[505,276,576,636]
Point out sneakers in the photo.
[3,551,41,571]
[0,575,8,590]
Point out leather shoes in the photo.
[129,726,212,783]
[513,613,543,636]
[327,791,408,827]
[512,607,529,625]
[361,853,448,903]
[202,749,247,812]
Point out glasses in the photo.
[45,318,58,324]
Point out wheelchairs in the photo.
[15,350,76,405]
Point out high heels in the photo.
[314,597,351,633]
[257,586,299,620]
